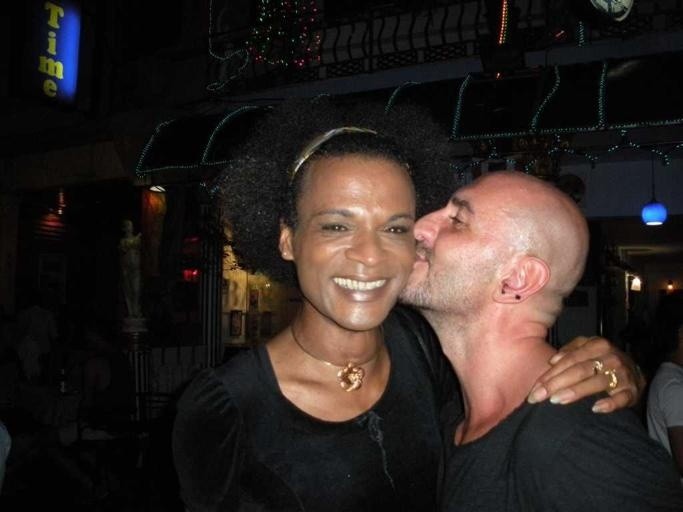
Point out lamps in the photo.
[640,149,668,228]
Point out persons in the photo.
[646,292,683,477]
[16,292,61,385]
[398,171,683,512]
[173,127,651,512]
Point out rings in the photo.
[592,359,602,374]
[605,371,619,391]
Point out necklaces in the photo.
[291,322,386,391]
